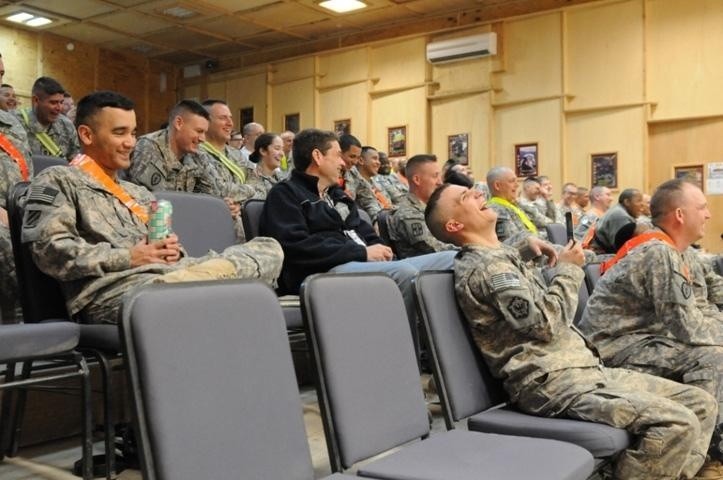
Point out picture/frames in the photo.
[334,119,351,138]
[675,165,704,192]
[447,133,469,166]
[283,112,301,133]
[388,125,406,157]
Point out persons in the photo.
[578,176,723,468]
[483,166,590,267]
[122,100,225,200]
[224,122,297,192]
[424,185,718,478]
[195,98,266,244]
[19,92,283,468]
[257,128,461,365]
[1,57,82,324]
[337,134,476,253]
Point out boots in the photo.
[142,259,240,283]
[698,455,720,480]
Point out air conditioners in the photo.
[426,32,498,66]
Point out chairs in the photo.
[2,155,317,480]
[547,223,568,246]
[585,264,604,290]
[412,270,629,455]
[545,266,590,326]
[379,208,395,237]
[299,272,594,479]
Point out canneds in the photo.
[147,198,172,247]
